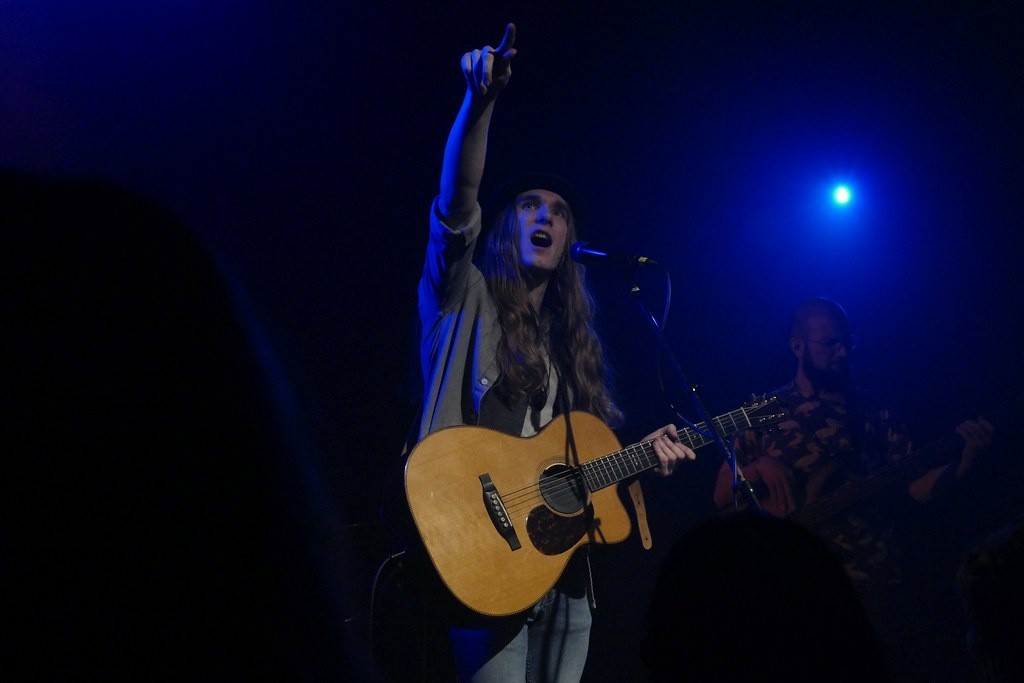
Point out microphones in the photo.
[571,240,668,272]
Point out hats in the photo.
[504,167,583,207]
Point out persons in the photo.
[415,23,696,683]
[713,299,993,597]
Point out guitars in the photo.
[784,431,964,529]
[388,392,784,629]
